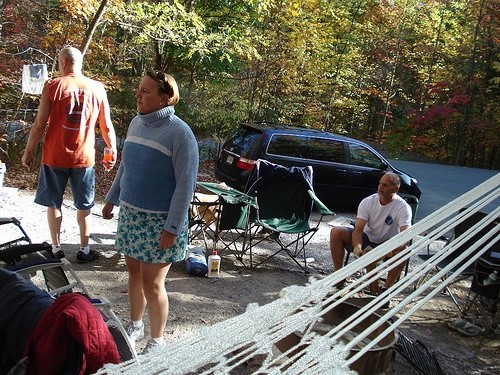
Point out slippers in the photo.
[447,315,486,336]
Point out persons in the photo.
[102,69,200,356]
[21,46,117,264]
[330,173,412,311]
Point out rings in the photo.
[168,245,172,248]
[22,163,24,164]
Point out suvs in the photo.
[214,124,421,214]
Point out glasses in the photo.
[154,70,169,94]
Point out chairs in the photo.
[0,217,137,375]
[188,158,336,272]
[343,193,500,317]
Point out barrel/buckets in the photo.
[269,298,400,375]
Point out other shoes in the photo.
[139,338,165,357]
[55,249,65,261]
[76,247,99,263]
[380,286,391,311]
[125,323,144,342]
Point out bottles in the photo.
[104,148,112,171]
[483,271,498,285]
[207,251,221,278]
